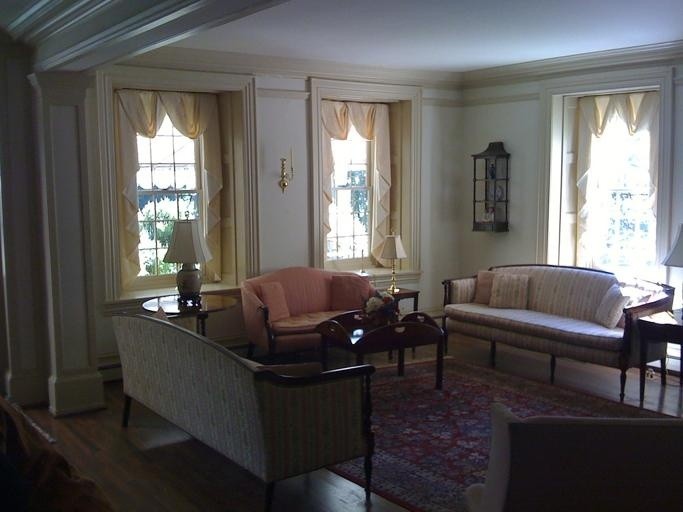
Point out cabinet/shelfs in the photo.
[471,141,510,232]
[661,222,683,324]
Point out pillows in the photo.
[474,270,529,309]
[481,397,523,512]
[331,274,370,311]
[595,284,652,329]
[256,282,290,321]
[151,307,169,322]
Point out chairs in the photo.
[466,416,683,512]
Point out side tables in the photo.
[141,294,238,338]
[637,307,683,401]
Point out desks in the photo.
[379,286,420,361]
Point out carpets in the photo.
[320,355,683,512]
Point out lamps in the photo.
[380,234,407,293]
[163,211,213,309]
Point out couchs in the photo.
[439,263,675,402]
[240,266,396,363]
[111,313,377,512]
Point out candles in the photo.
[291,146,293,168]
